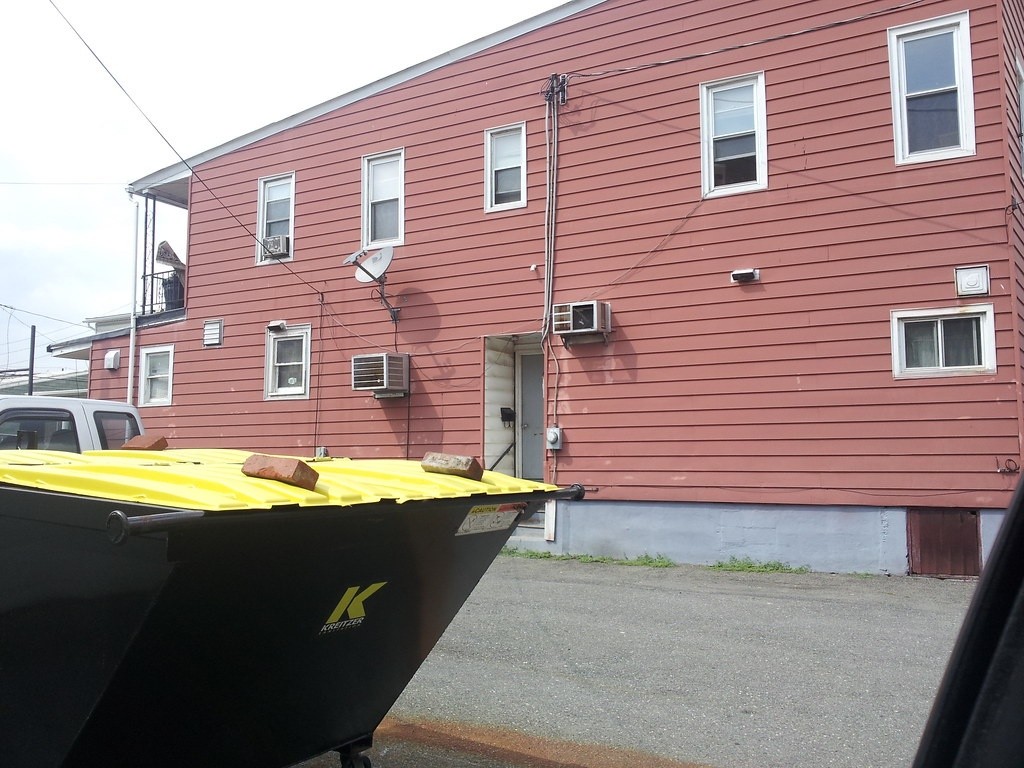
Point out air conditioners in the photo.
[552,301,612,335]
[352,353,409,398]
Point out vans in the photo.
[0,393,146,453]
